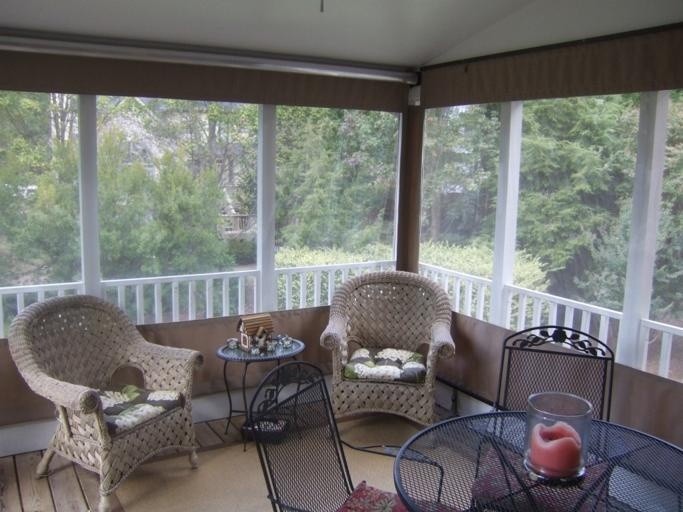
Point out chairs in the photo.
[6,293,205,512]
[248,362,457,510]
[315,271,456,450]
[474,325,614,512]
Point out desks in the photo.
[391,409,682,512]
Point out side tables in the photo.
[216,335,307,454]
